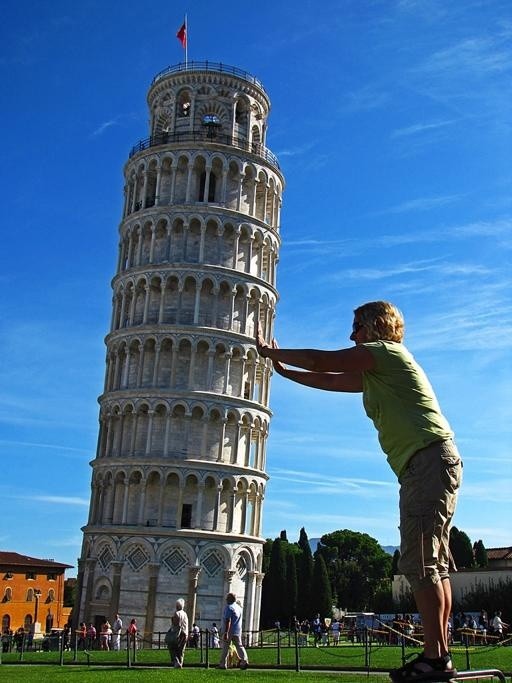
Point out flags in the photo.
[176,22,186,49]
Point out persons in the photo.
[369,609,510,646]
[256,300,463,683]
[0,593,250,670]
[275,614,345,646]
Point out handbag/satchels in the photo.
[165,629,181,644]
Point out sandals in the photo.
[390,651,456,683]
